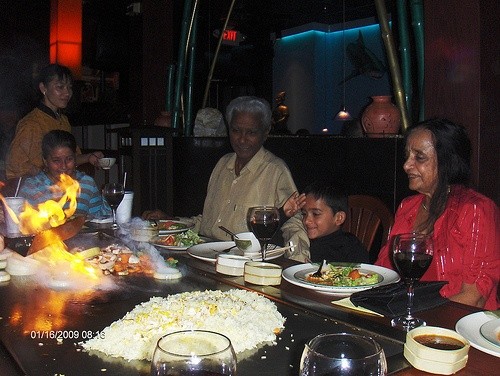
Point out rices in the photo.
[76,287,294,359]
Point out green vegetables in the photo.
[325,264,379,288]
[171,222,203,248]
[134,248,179,267]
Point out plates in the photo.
[479,319,500,348]
[187,241,285,262]
[282,262,401,297]
[455,311,500,359]
[152,220,196,251]
[293,266,384,288]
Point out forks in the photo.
[209,246,237,253]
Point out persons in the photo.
[4,62,106,180]
[140,94,312,266]
[13,130,113,221]
[370,117,500,314]
[259,177,372,264]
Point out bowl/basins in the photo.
[243,262,283,286]
[98,158,117,169]
[234,232,261,255]
[215,254,251,277]
[403,325,470,375]
[132,227,158,242]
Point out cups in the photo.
[115,190,134,225]
[151,329,238,376]
[1,197,26,238]
[298,332,388,376]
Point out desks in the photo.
[171,136,420,223]
[0,215,500,376]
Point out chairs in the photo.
[340,195,393,253]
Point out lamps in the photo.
[49,0,84,74]
[333,0,354,121]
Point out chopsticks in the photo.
[251,245,297,258]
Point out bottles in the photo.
[155,112,171,127]
[361,95,400,139]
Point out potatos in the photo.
[114,252,154,274]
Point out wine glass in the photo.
[390,233,434,331]
[249,207,280,262]
[104,183,125,230]
